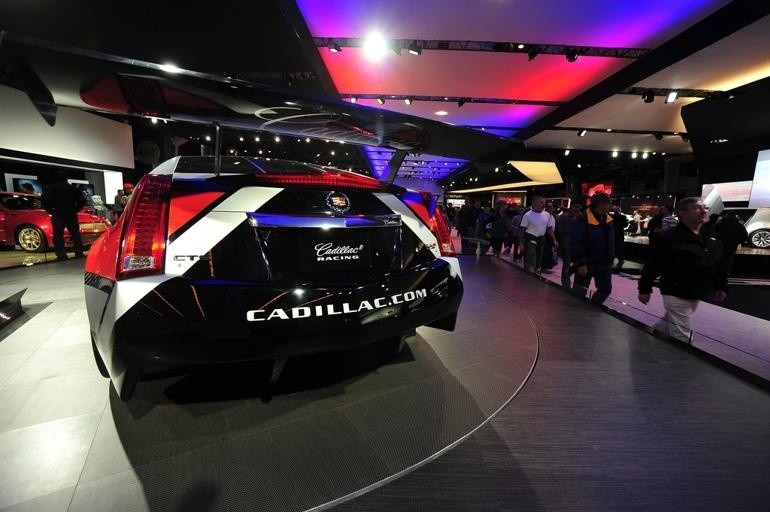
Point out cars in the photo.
[0,190,114,253]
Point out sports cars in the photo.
[0,29,529,404]
[693,184,770,249]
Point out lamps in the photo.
[351,94,415,106]
[328,40,342,53]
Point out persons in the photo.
[21,183,37,194]
[449,192,749,305]
[78,184,88,198]
[41,169,86,262]
[114,190,133,219]
[637,195,730,346]
